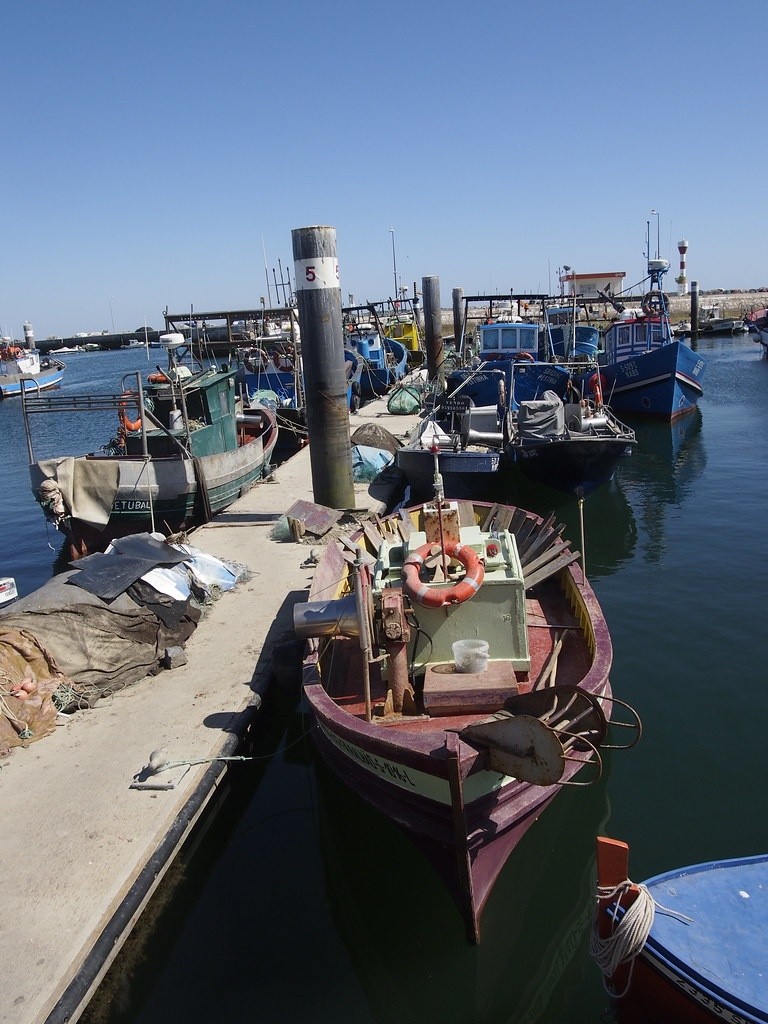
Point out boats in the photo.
[120,340,147,349]
[742,301,768,346]
[48,345,86,356]
[0,336,67,399]
[83,343,104,351]
[140,256,755,495]
[590,835,768,1024]
[11,364,279,544]
[294,441,642,945]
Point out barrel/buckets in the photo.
[452,639,489,674]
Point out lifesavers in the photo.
[244,347,268,375]
[400,540,485,608]
[146,372,170,384]
[513,350,537,365]
[14,347,23,360]
[593,382,602,408]
[640,288,671,320]
[117,389,144,432]
[272,346,295,372]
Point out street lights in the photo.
[650,208,661,260]
[389,226,397,298]
[642,220,651,261]
